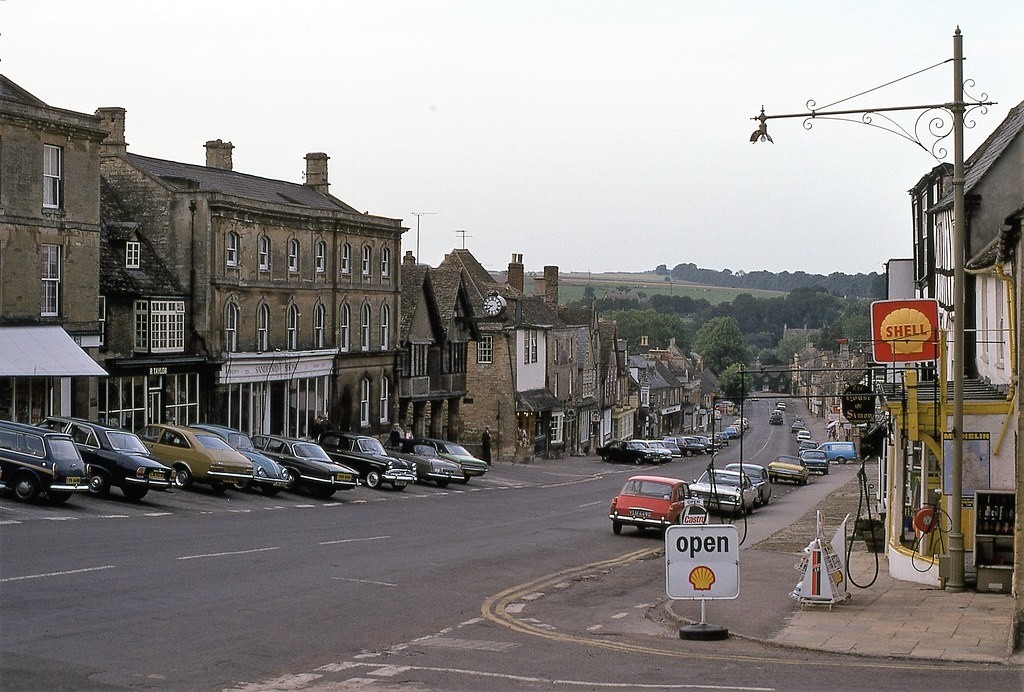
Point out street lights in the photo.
[749,23,998,591]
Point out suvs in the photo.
[317,431,416,491]
[0,419,90,505]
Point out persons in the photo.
[482,426,493,466]
[388,422,414,447]
[311,414,332,444]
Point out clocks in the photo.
[481,295,507,317]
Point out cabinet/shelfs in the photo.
[971,489,1018,570]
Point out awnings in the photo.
[0,324,110,379]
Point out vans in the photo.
[798,442,858,463]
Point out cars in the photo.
[596,418,749,466]
[752,396,759,401]
[135,424,253,495]
[248,434,362,498]
[719,463,772,506]
[715,401,740,419]
[768,398,786,425]
[767,454,810,486]
[792,415,819,458]
[187,423,290,496]
[801,450,830,474]
[32,416,178,501]
[608,475,694,540]
[383,437,488,487]
[688,469,759,517]
